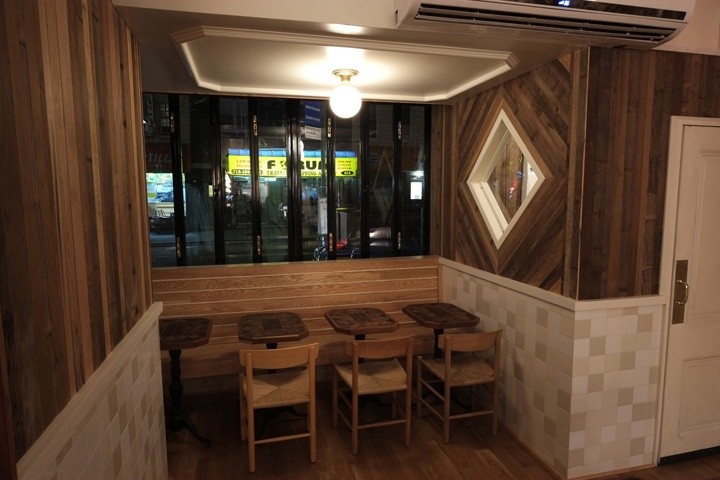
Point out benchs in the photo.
[151,253,440,381]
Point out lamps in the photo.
[329,68,363,119]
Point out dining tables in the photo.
[238,312,308,440]
[324,307,400,418]
[403,302,480,412]
[159,317,212,445]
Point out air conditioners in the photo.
[394,1,697,54]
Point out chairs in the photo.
[417,329,504,444]
[331,334,415,455]
[239,342,319,473]
